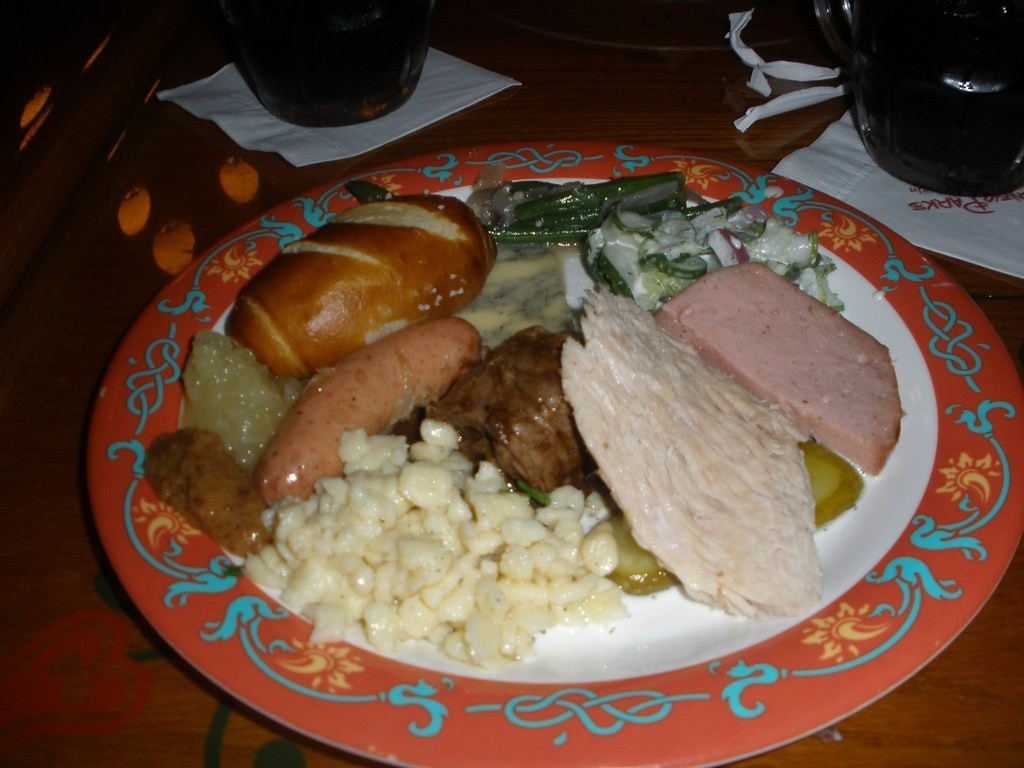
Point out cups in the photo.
[812,0,1024,197]
[217,0,436,129]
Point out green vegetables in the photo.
[345,173,821,309]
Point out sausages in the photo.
[253,318,482,508]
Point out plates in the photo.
[83,140,1021,768]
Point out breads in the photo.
[225,195,497,379]
